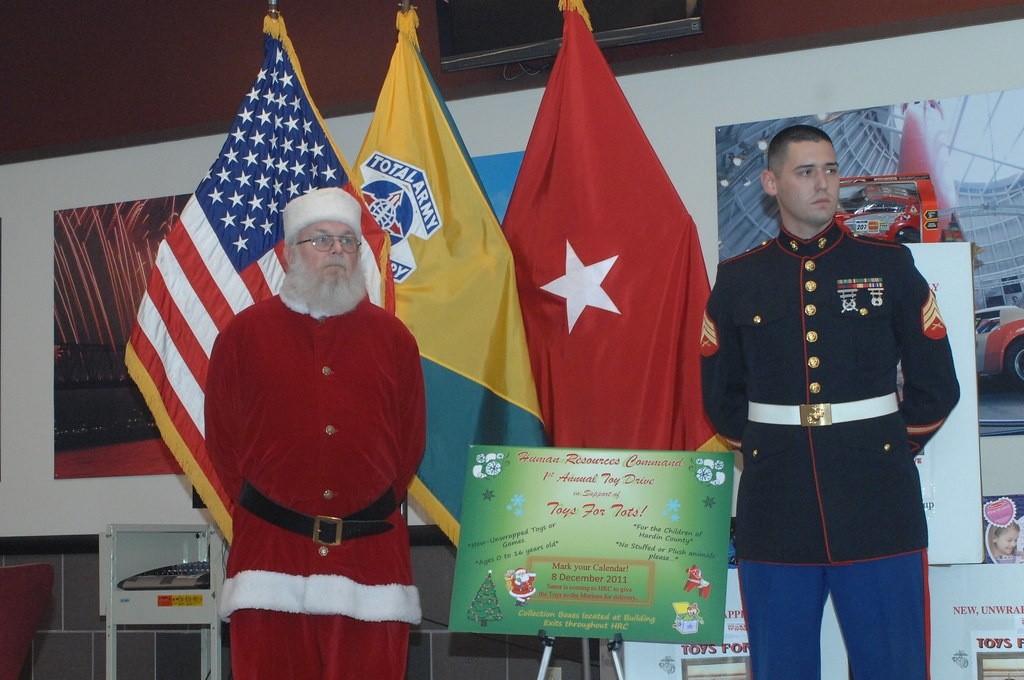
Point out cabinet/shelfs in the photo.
[105,524,232,680]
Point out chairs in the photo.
[0,563,55,680]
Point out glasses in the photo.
[293,235,362,254]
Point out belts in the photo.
[747,391,901,426]
[239,479,398,546]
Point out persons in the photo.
[699,125,960,680]
[204,188,427,680]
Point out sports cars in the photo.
[975,293,1024,393]
[832,183,922,244]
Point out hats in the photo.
[283,187,362,245]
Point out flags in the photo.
[122,14,393,549]
[500,0,733,453]
[353,9,547,544]
